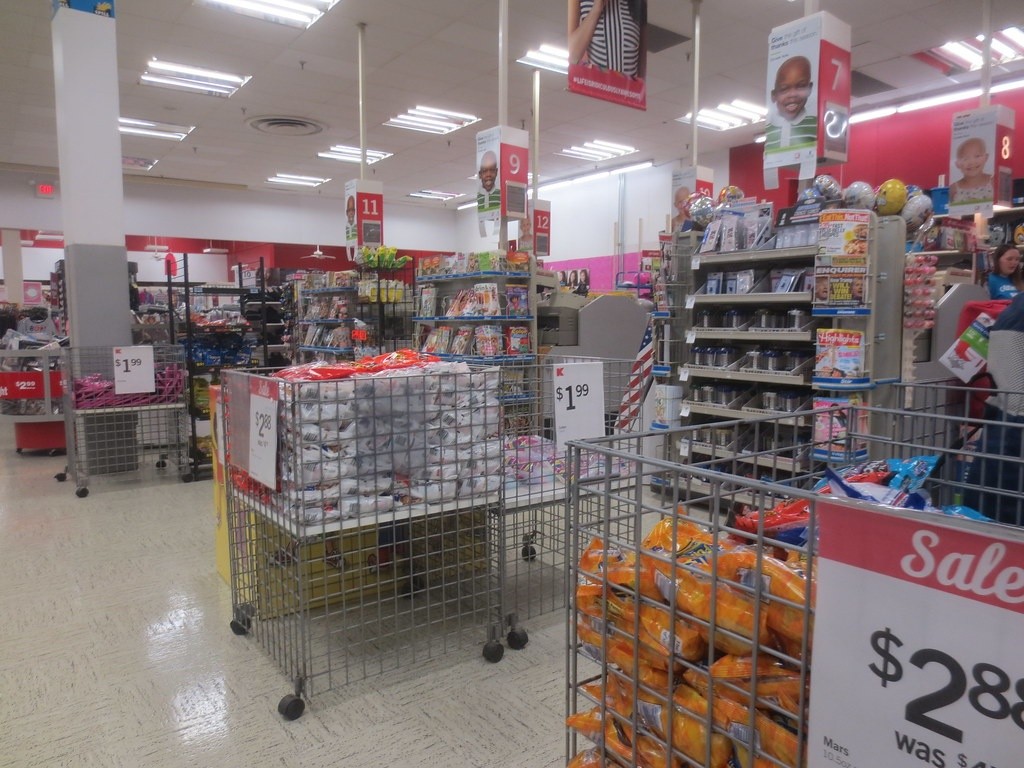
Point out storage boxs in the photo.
[930,187,949,216]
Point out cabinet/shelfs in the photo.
[613,209,906,509]
[166,250,558,482]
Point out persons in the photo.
[990,261,1024,332]
[672,186,690,233]
[850,278,863,304]
[814,277,830,302]
[568,0,648,78]
[567,270,578,286]
[983,244,1024,300]
[579,268,588,285]
[518,216,534,250]
[949,138,994,203]
[346,195,358,241]
[559,271,567,286]
[476,151,500,209]
[763,55,818,151]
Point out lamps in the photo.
[20,231,34,247]
[202,240,229,253]
[144,236,169,251]
